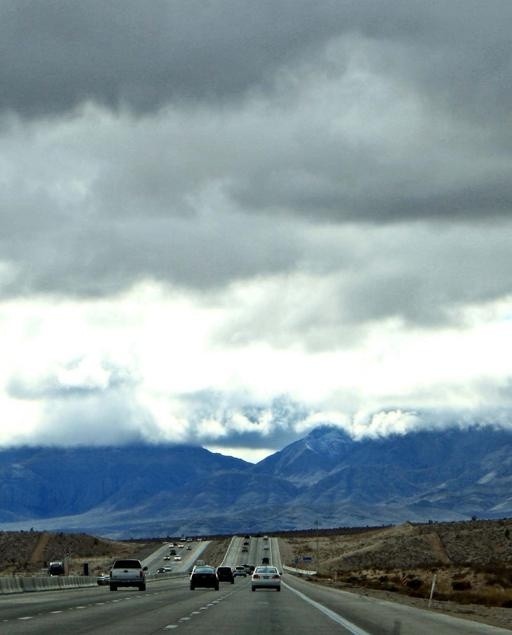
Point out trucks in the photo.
[49,561,69,578]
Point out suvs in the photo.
[111,558,148,591]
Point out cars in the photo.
[189,564,219,590]
[233,564,251,577]
[157,537,194,574]
[251,565,282,592]
[241,535,250,551]
[217,566,234,584]
[260,557,269,564]
[263,545,268,549]
[97,574,110,585]
[263,536,268,540]
